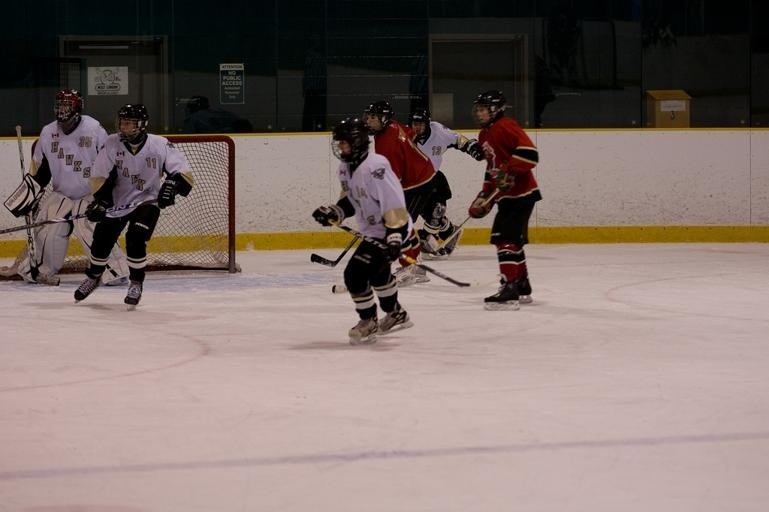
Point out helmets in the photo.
[53,88,149,143]
[184,95,209,118]
[331,89,507,164]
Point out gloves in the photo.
[469,191,495,218]
[382,232,403,265]
[312,204,345,227]
[84,198,109,222]
[158,177,181,209]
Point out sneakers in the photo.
[349,227,532,339]
[74,273,142,305]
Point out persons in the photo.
[470,91,541,312]
[312,115,413,347]
[410,110,482,257]
[3,87,131,286]
[364,102,433,287]
[74,102,194,311]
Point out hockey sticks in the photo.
[15,125,60,286]
[426,189,500,256]
[328,218,497,287]
[312,235,361,266]
